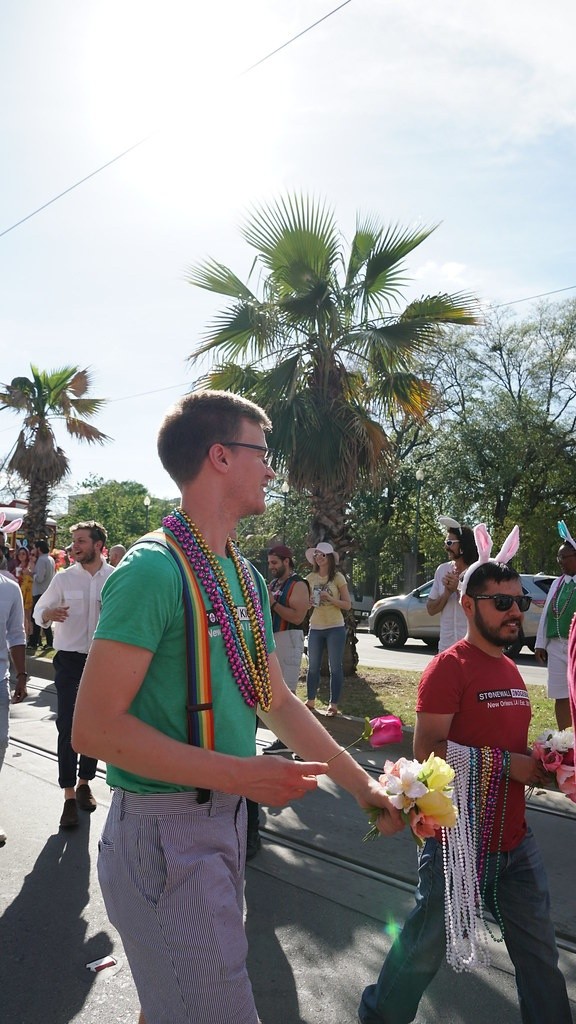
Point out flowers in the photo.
[361,747,460,845]
[315,712,405,766]
[523,727,576,805]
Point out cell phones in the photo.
[313,590,321,608]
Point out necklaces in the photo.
[442,739,503,974]
[551,575,576,645]
[270,571,294,601]
[163,506,273,713]
[481,748,511,943]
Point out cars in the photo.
[368,573,560,660]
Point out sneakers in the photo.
[77,784,97,810]
[295,756,302,760]
[263,739,292,753]
[60,799,79,827]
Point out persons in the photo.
[426,516,479,654]
[28,547,38,570]
[12,539,34,649]
[357,522,572,1024]
[0,512,28,842]
[71,388,408,1024]
[5,540,20,573]
[32,520,116,829]
[109,544,126,567]
[534,520,576,732]
[50,549,67,573]
[262,545,311,761]
[302,542,352,717]
[27,541,54,651]
[65,542,74,567]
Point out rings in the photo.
[447,581,449,584]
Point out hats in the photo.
[269,546,293,558]
[305,543,339,565]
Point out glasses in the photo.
[557,553,574,560]
[474,594,533,612]
[313,553,327,558]
[445,540,459,547]
[221,442,276,469]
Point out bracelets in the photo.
[271,601,278,609]
[16,672,28,679]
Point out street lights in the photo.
[281,480,289,547]
[143,496,151,533]
[415,468,424,557]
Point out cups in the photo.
[15,568,22,576]
[312,589,321,607]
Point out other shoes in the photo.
[325,708,337,717]
[26,645,33,649]
[44,644,53,650]
[305,704,313,709]
